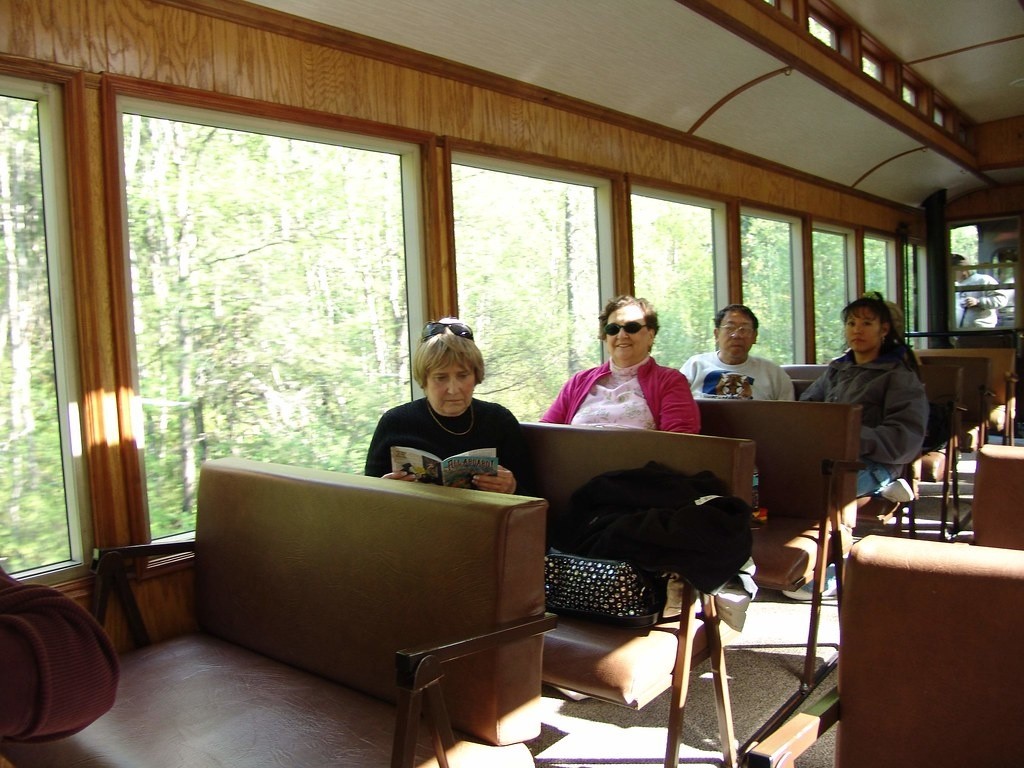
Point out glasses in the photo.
[421,321,473,340]
[718,326,756,337]
[604,322,649,336]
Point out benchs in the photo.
[0,338,1024,768]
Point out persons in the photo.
[949,252,1016,330]
[679,303,796,603]
[779,290,984,602]
[538,294,702,434]
[363,317,543,498]
[0,559,121,745]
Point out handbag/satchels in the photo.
[542,553,661,628]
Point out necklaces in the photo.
[427,399,475,435]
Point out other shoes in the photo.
[783,589,814,601]
